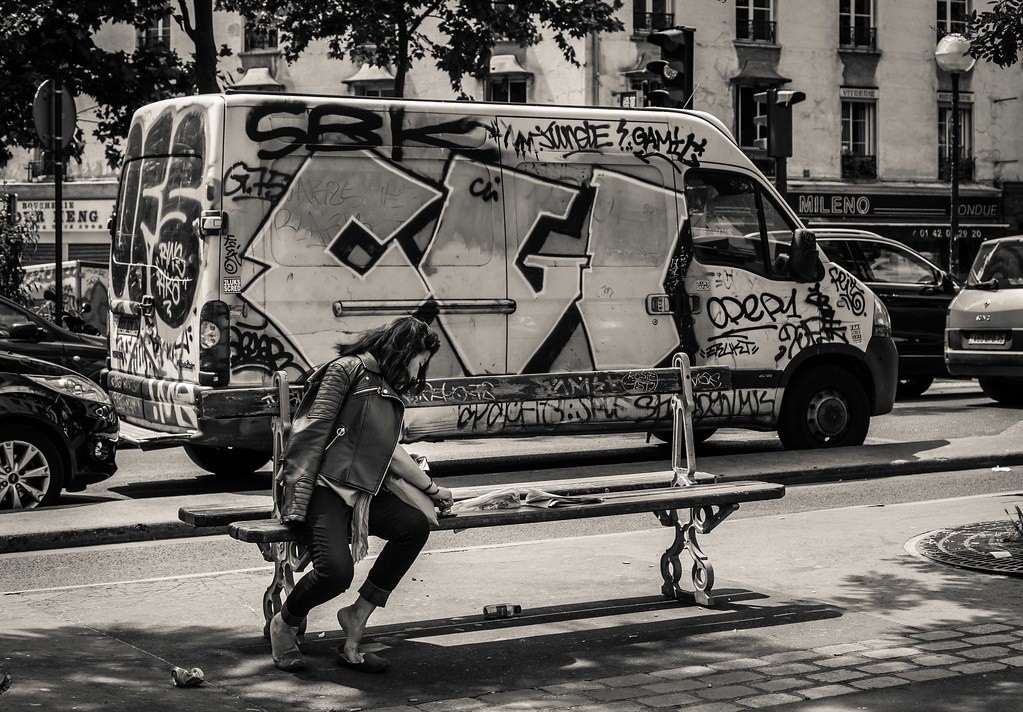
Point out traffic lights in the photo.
[754,87,805,159]
[645,25,695,109]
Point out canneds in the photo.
[172,667,206,688]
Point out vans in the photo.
[100,92,897,477]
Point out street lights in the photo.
[934,34,977,281]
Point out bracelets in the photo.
[422,477,433,491]
[428,488,439,495]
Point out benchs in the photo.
[179,352,785,657]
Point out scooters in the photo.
[42,290,91,333]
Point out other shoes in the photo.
[335,651,389,672]
[272,645,304,669]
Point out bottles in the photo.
[483,603,521,617]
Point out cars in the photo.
[0,295,120,513]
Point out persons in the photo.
[269,315,454,674]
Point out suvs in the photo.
[737,226,1023,405]
